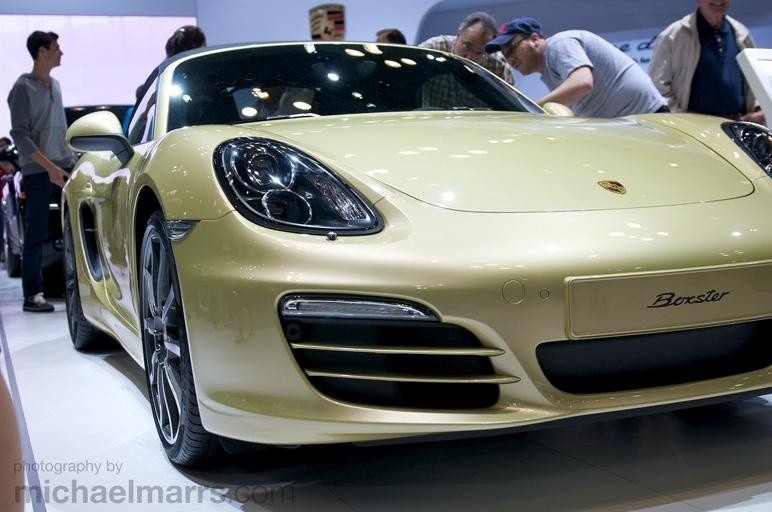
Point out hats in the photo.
[484,17,546,54]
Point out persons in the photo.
[375,29,406,45]
[122,84,144,138]
[484,18,671,119]
[649,1,765,124]
[165,25,207,59]
[7,31,78,312]
[417,12,516,108]
[0,138,20,175]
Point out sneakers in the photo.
[22,290,55,312]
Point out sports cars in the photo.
[60,38,772,474]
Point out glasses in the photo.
[503,33,532,60]
[460,30,488,59]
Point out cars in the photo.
[1,104,136,302]
